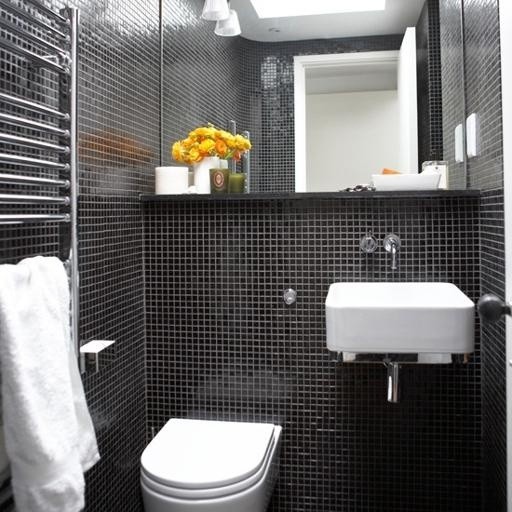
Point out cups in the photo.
[209,168,231,195]
[228,172,246,194]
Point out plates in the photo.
[371,173,443,192]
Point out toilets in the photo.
[139,418,283,512]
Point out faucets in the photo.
[383,233,402,270]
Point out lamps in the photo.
[213,1,241,37]
[201,0,229,21]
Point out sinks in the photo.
[325,282,475,355]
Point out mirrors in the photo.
[158,0,444,194]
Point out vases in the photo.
[192,156,219,194]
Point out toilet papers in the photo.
[155,167,188,195]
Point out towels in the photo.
[0,255,104,512]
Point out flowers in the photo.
[170,126,233,166]
